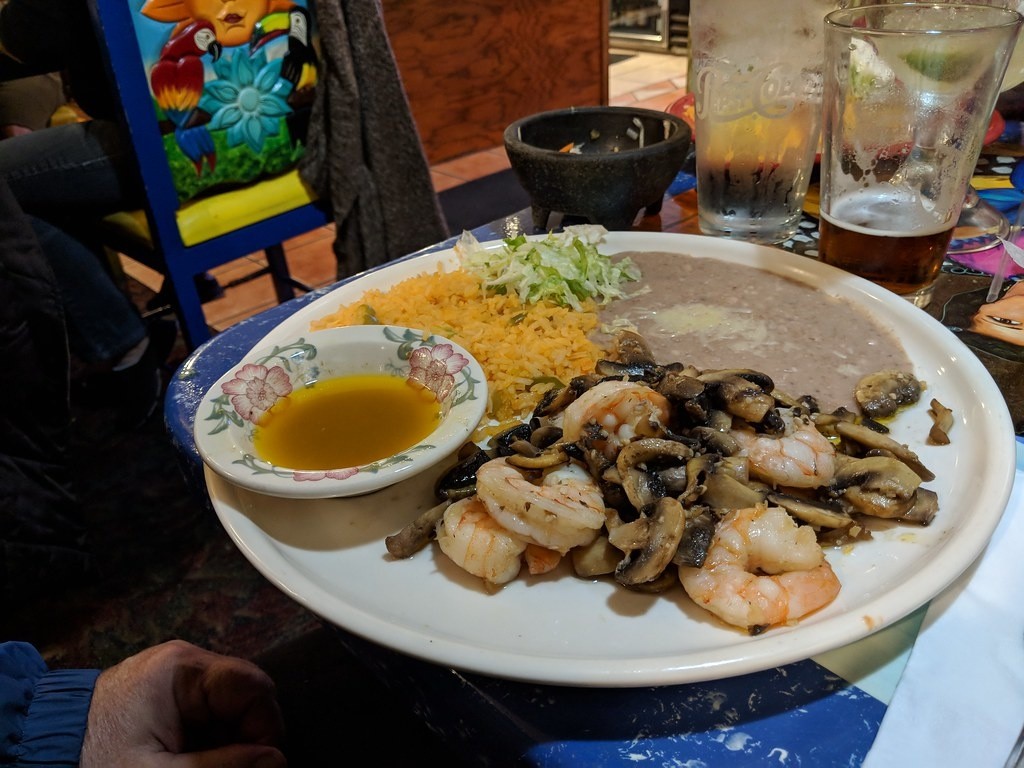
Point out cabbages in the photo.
[453,221,652,310]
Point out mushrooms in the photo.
[383,330,956,593]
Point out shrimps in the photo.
[437,381,841,635]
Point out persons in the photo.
[0,0,289,768]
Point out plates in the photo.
[204,230,1016,689]
[667,95,1005,167]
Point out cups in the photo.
[690,0,839,245]
[818,5,1024,309]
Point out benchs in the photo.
[50,0,451,358]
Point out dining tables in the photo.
[165,124,1024,768]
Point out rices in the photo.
[303,259,612,443]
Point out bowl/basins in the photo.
[501,106,691,232]
[194,322,489,501]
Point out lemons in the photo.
[895,38,992,82]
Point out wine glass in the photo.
[836,0,1023,254]
